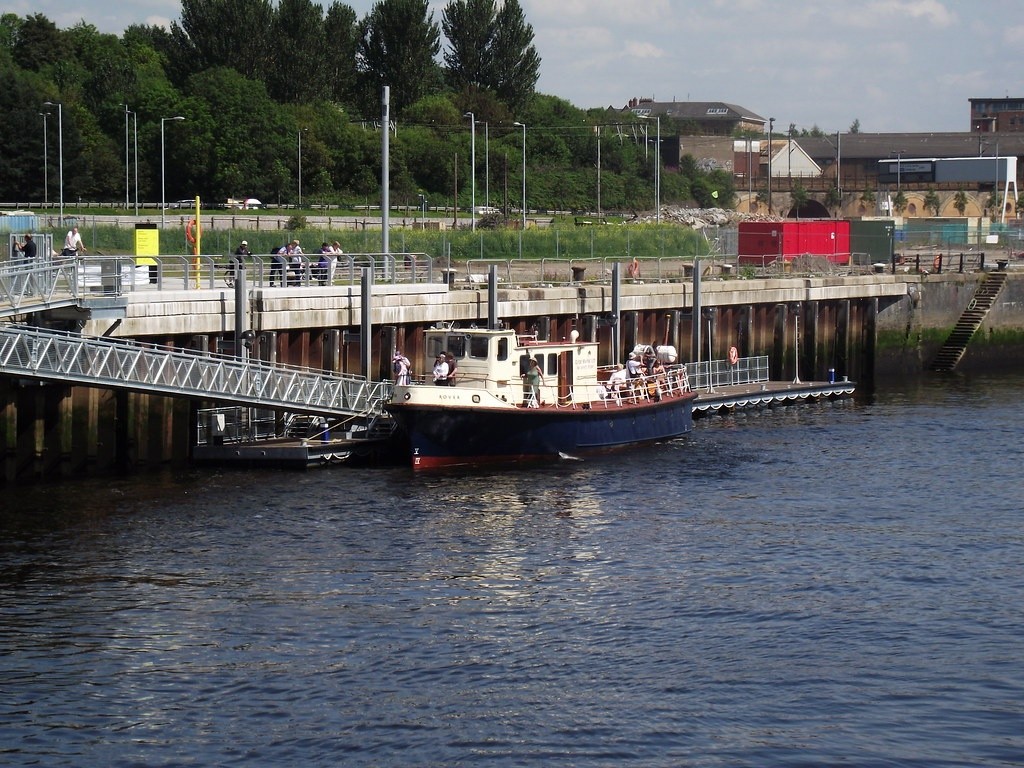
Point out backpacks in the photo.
[270,247,289,259]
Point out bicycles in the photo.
[59,248,85,273]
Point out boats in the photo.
[385,326,697,471]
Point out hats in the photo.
[530,358,538,363]
[242,240,248,246]
[629,352,636,359]
[655,360,661,364]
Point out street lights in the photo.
[638,115,660,224]
[466,111,475,233]
[892,149,906,192]
[39,112,51,203]
[161,116,185,229]
[475,120,488,215]
[299,128,308,209]
[649,140,663,206]
[126,111,138,215]
[513,122,526,229]
[119,103,129,210]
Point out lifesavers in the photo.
[187,220,202,243]
[628,261,639,275]
[729,347,738,365]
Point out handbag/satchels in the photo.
[394,361,401,374]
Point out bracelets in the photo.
[81,246,85,251]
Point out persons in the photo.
[229,240,253,288]
[520,357,546,407]
[606,343,670,404]
[269,240,344,287]
[64,226,88,256]
[433,351,459,387]
[393,351,411,386]
[48,243,60,260]
[15,233,37,296]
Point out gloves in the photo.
[248,251,253,255]
